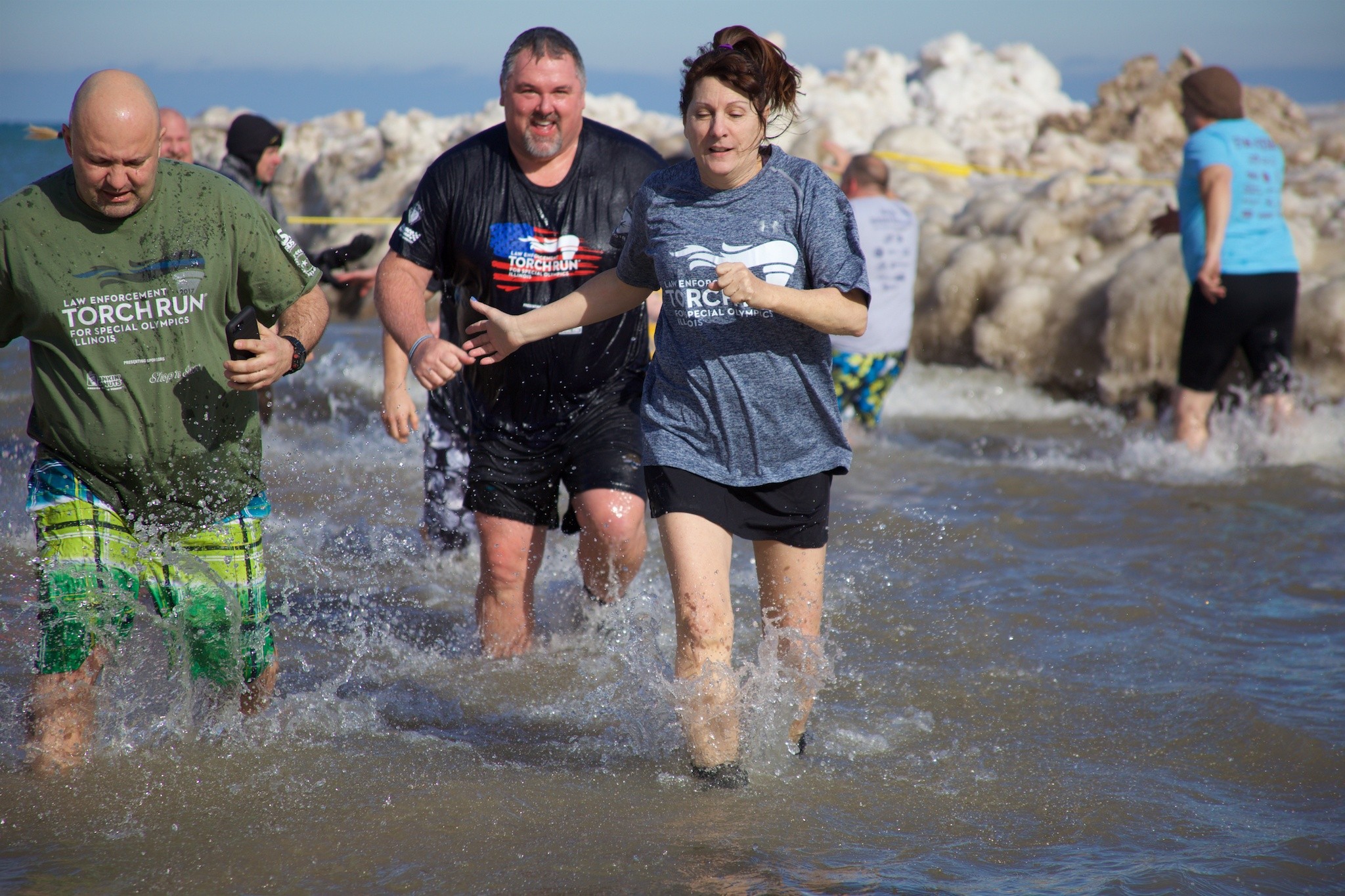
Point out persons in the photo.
[312,233,375,324]
[379,272,474,564]
[1147,67,1298,452]
[830,153,918,439]
[0,67,330,781]
[218,113,374,427]
[374,24,665,661]
[461,25,871,789]
[158,109,248,192]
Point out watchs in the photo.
[280,336,307,377]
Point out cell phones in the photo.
[226,307,260,361]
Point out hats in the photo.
[1182,67,1243,119]
[225,115,283,169]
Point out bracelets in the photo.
[408,335,434,362]
